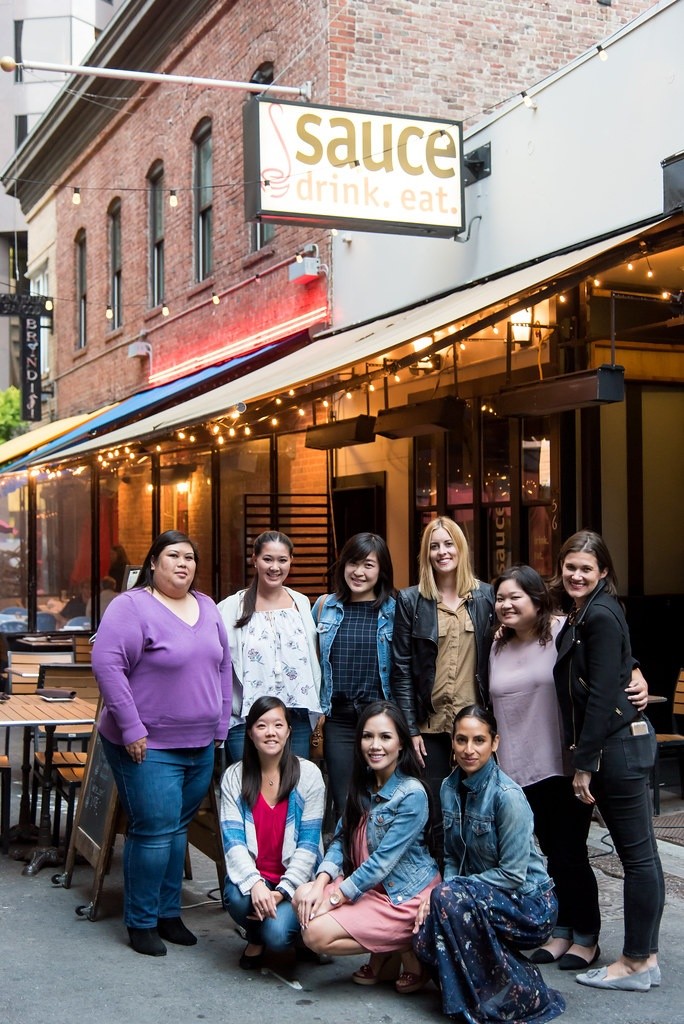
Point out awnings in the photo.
[0,402,120,464]
[30,214,677,465]
[0,329,305,474]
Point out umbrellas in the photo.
[0,520,13,532]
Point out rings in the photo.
[260,908,264,910]
[575,794,580,796]
[426,902,430,904]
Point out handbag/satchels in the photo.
[310,714,324,758]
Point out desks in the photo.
[1,692,109,876]
[16,639,73,650]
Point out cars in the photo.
[0,607,93,633]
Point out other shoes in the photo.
[575,965,650,992]
[558,943,600,970]
[157,917,197,946]
[236,928,265,970]
[587,965,660,986]
[528,939,574,964]
[293,938,334,965]
[126,926,167,956]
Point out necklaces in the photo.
[266,776,273,785]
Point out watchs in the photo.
[330,890,342,908]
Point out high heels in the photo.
[396,958,435,992]
[352,951,402,985]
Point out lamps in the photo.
[408,335,440,374]
[135,446,149,464]
[503,307,534,347]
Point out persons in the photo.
[61,581,91,618]
[85,576,119,620]
[489,565,648,969]
[109,545,130,592]
[392,518,494,877]
[415,705,565,1024]
[220,697,442,994]
[312,533,395,826]
[91,530,231,958]
[494,533,665,992]
[216,531,321,759]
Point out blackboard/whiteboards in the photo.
[72,694,119,875]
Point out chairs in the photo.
[0,631,103,886]
[654,666,684,817]
